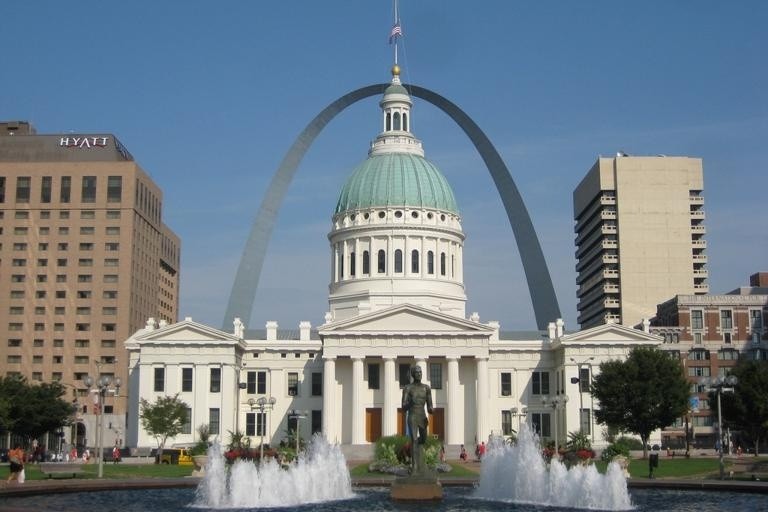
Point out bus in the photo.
[661,431,686,456]
[152,448,195,464]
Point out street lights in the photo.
[93,358,118,463]
[684,344,706,456]
[570,356,595,435]
[699,374,738,480]
[288,409,308,454]
[511,407,528,427]
[543,395,569,454]
[85,375,122,478]
[248,397,276,461]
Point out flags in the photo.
[388,22,403,44]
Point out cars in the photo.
[29,449,63,462]
[257,444,278,459]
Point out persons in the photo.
[478,441,486,458]
[439,446,446,463]
[112,444,119,464]
[4,442,24,485]
[474,445,480,459]
[401,365,436,480]
[736,445,742,455]
[28,442,90,464]
[714,439,720,453]
[459,444,466,461]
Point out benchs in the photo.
[41,464,84,479]
